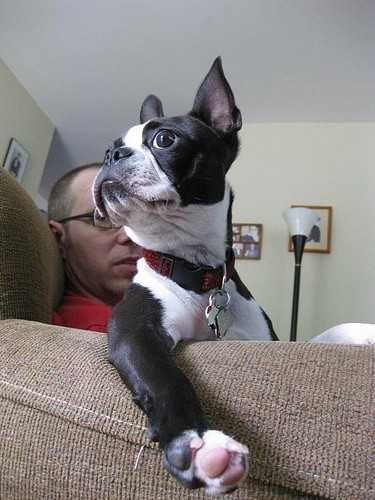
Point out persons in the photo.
[232,225,259,256]
[48,162,143,332]
[10,153,21,177]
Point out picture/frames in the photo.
[288,205,332,254]
[3,138,31,185]
[233,222,263,260]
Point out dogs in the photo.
[91,55,280,496]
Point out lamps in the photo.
[282,207,317,340]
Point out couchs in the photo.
[0,170,375,499]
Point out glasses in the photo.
[56,208,126,231]
